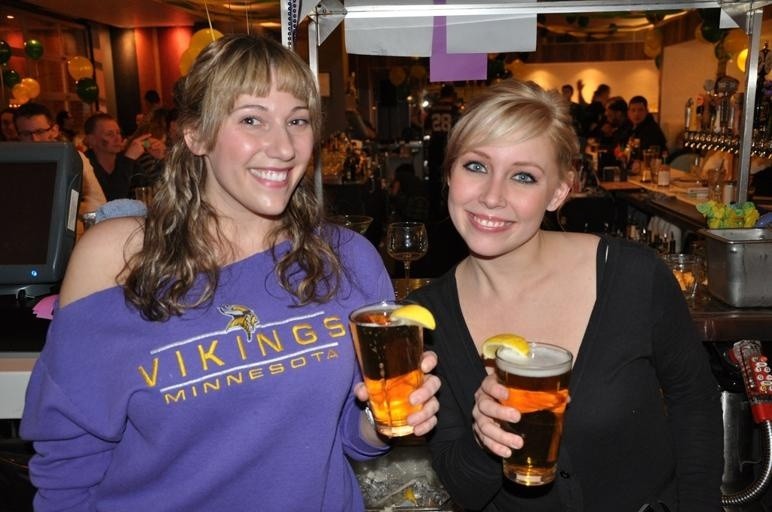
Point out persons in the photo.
[17,31,443,512]
[0,89,181,240]
[562,79,670,164]
[388,83,459,259]
[402,79,724,512]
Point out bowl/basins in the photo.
[334,214,374,234]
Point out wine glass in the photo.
[386,221,430,305]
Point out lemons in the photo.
[481,332,533,361]
[388,303,437,332]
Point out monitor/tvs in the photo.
[0,140,83,301]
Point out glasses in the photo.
[20,126,53,135]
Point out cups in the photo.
[135,186,153,207]
[708,168,726,203]
[494,340,574,486]
[662,254,702,300]
[350,301,427,438]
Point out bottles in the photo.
[657,151,670,190]
